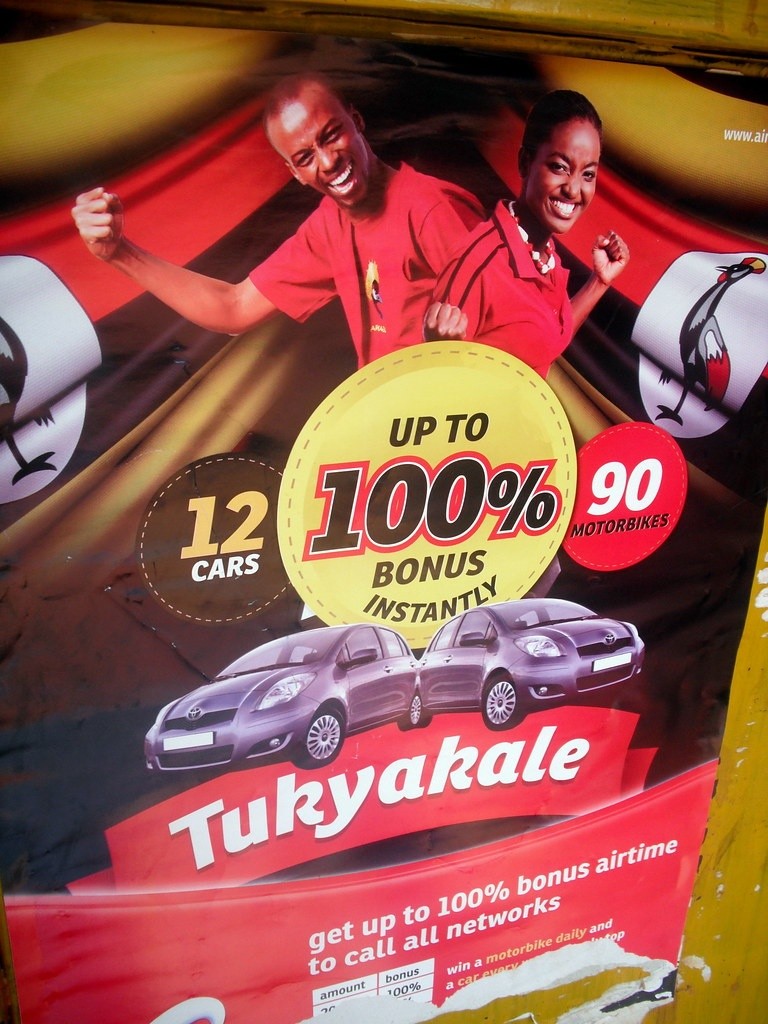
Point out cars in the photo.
[409,599,645,731]
[144,623,422,782]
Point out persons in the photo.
[72,73,630,376]
